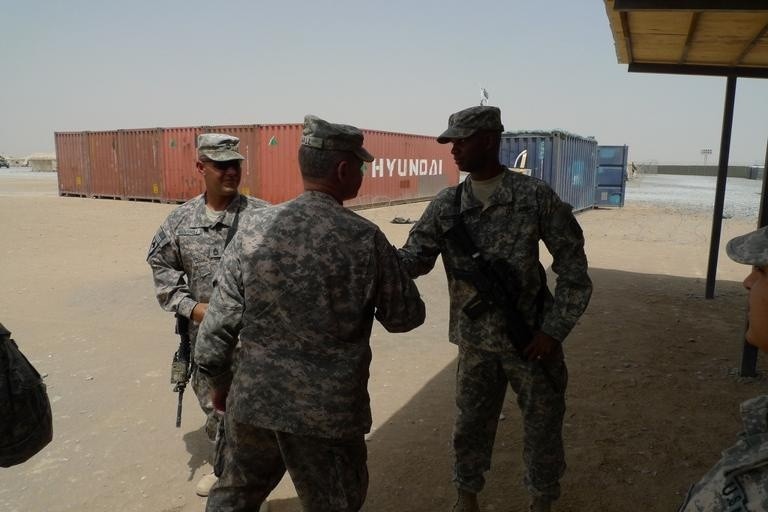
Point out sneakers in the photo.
[196,470,221,497]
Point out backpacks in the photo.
[0,322,54,468]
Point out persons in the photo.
[147,133,274,498]
[193,114,427,510]
[394,106,595,510]
[676,225,768,512]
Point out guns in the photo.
[441,221,561,394]
[172,315,190,427]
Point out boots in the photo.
[527,493,552,512]
[448,484,480,512]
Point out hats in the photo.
[301,113,374,163]
[726,225,768,266]
[197,133,246,163]
[437,105,504,144]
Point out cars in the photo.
[0,158,9,168]
